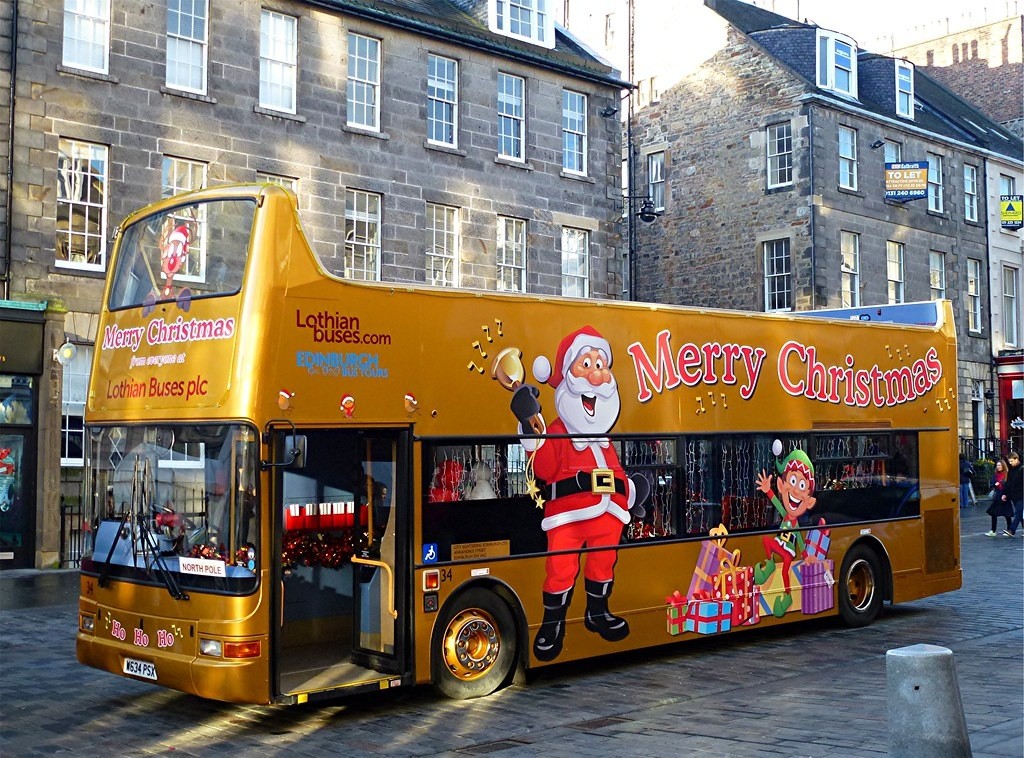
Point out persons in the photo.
[375,474,388,540]
[960,452,1024,538]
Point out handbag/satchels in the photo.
[962,466,973,477]
[988,488,997,502]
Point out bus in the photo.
[74,180,964,710]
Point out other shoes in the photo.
[1003,530,1014,537]
[974,501,978,505]
[984,530,997,536]
[1003,533,1010,536]
